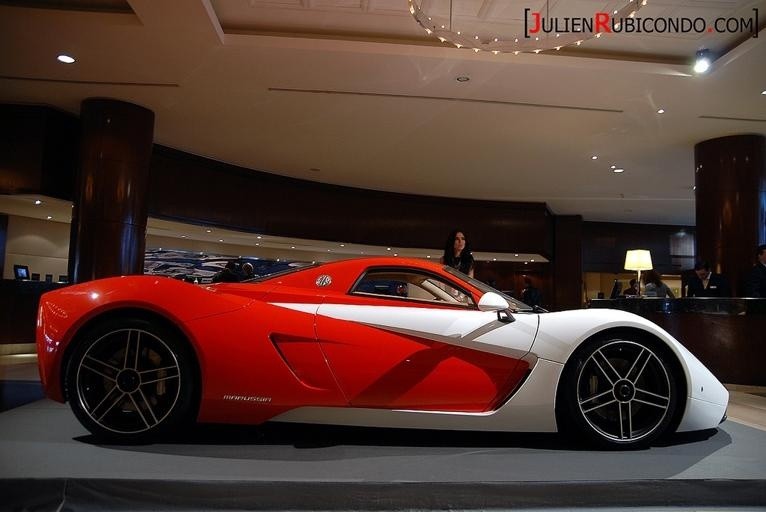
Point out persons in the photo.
[623,244,766,298]
[213,261,240,282]
[440,229,475,304]
[242,262,255,279]
[520,279,542,307]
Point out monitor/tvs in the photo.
[13,264,30,280]
[610,280,624,298]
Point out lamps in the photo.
[408,0,655,56]
[624,248,653,299]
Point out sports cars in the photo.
[36,257,730,450]
